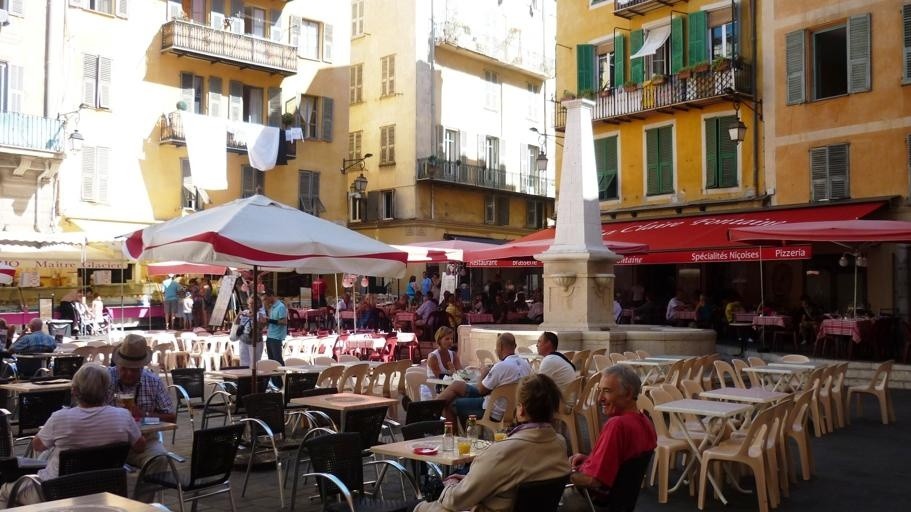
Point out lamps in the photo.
[727,93,767,146]
[339,152,374,191]
[57,111,84,140]
[536,133,565,172]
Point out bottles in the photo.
[442,421,454,450]
[466,415,477,445]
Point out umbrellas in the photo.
[117,191,413,397]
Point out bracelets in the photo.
[145,410,152,418]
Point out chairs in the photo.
[713,357,766,387]
[781,388,814,480]
[18,390,71,440]
[554,376,584,454]
[8,443,131,507]
[33,357,83,378]
[564,348,606,372]
[397,400,445,492]
[681,354,720,389]
[809,362,848,437]
[285,373,317,402]
[518,347,533,355]
[282,328,422,365]
[577,372,602,448]
[560,449,656,512]
[316,359,436,401]
[287,307,331,330]
[303,432,423,512]
[18,345,54,382]
[475,349,495,368]
[424,311,456,343]
[238,393,320,510]
[846,360,894,424]
[149,326,242,372]
[284,406,388,510]
[473,383,518,436]
[73,339,120,367]
[782,355,809,364]
[136,422,245,510]
[591,349,651,373]
[637,380,736,503]
[208,376,269,395]
[168,368,229,445]
[698,400,792,512]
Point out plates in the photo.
[414,447,437,454]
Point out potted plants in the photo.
[558,56,734,106]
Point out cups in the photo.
[456,437,472,458]
[118,393,133,417]
[493,431,507,444]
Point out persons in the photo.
[609,277,829,352]
[7,316,58,359]
[56,270,291,371]
[326,267,545,346]
[529,331,581,414]
[100,335,179,505]
[1,361,147,509]
[409,372,570,512]
[559,363,659,511]
[427,325,466,398]
[438,332,533,436]
[0,318,16,354]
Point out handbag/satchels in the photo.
[422,460,447,503]
[229,324,240,342]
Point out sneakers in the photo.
[402,395,414,412]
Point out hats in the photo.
[111,333,153,369]
